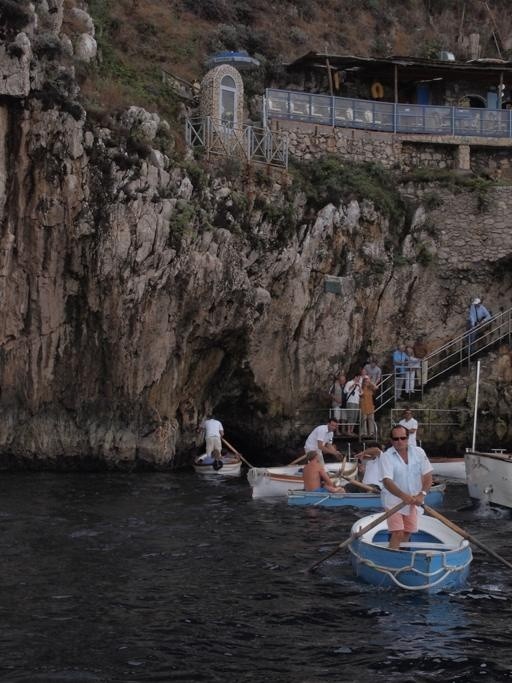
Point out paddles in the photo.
[308,503,405,569]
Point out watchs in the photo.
[419,491,427,496]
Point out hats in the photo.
[472,298,481,305]
[307,450,317,460]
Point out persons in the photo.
[303,417,340,472]
[378,423,433,551]
[501,96,512,129]
[469,298,490,352]
[328,345,420,495]
[198,450,238,466]
[199,411,225,456]
[303,450,346,494]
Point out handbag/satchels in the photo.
[396,368,401,377]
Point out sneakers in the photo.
[334,431,375,436]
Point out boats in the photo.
[192,452,242,477]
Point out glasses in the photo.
[391,436,407,442]
